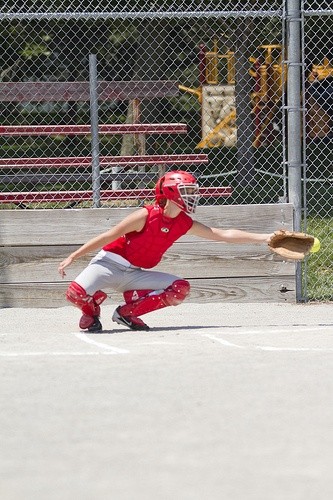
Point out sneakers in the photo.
[112,306,154,332]
[87,317,102,332]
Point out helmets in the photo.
[157,171,200,215]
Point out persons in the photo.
[57,171,321,331]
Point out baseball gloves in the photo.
[266,230,314,261]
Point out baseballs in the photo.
[309,237,321,253]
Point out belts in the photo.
[100,249,141,269]
